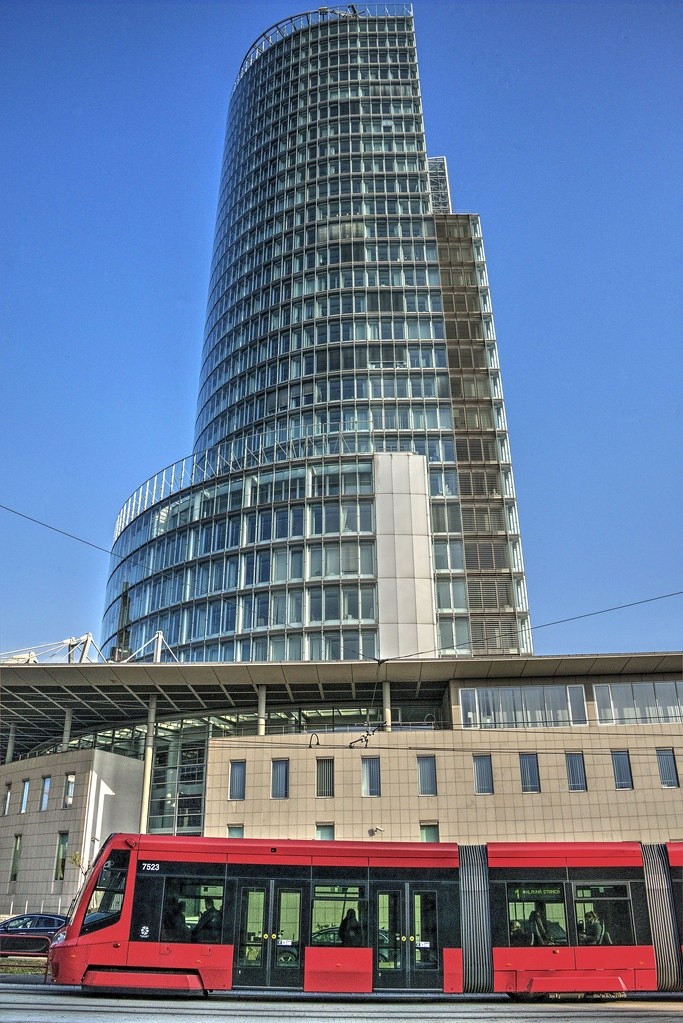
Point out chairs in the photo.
[597,920,606,945]
[208,905,223,937]
[527,910,560,947]
[164,896,178,934]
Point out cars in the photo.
[83,906,123,925]
[0,912,70,958]
[255,925,392,968]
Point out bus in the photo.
[42,830,683,1002]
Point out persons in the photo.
[510,919,528,946]
[171,901,186,929]
[578,912,602,944]
[338,909,362,947]
[190,898,216,941]
